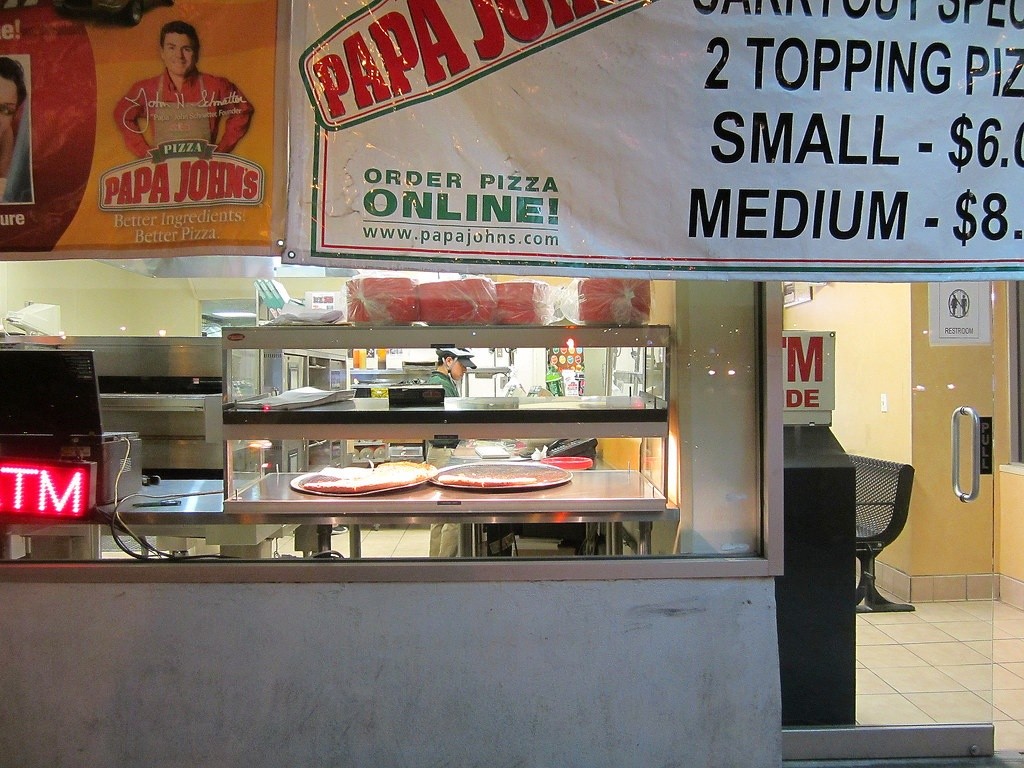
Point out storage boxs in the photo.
[388,383,446,408]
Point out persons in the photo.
[113,21,254,158]
[426,348,477,557]
[0,57,27,203]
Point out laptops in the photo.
[0,349,139,441]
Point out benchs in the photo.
[849,453,915,613]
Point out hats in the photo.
[458,357,477,369]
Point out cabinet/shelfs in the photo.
[220,324,673,515]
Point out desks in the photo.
[5,479,301,560]
[452,439,624,558]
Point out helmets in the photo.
[436,348,475,358]
